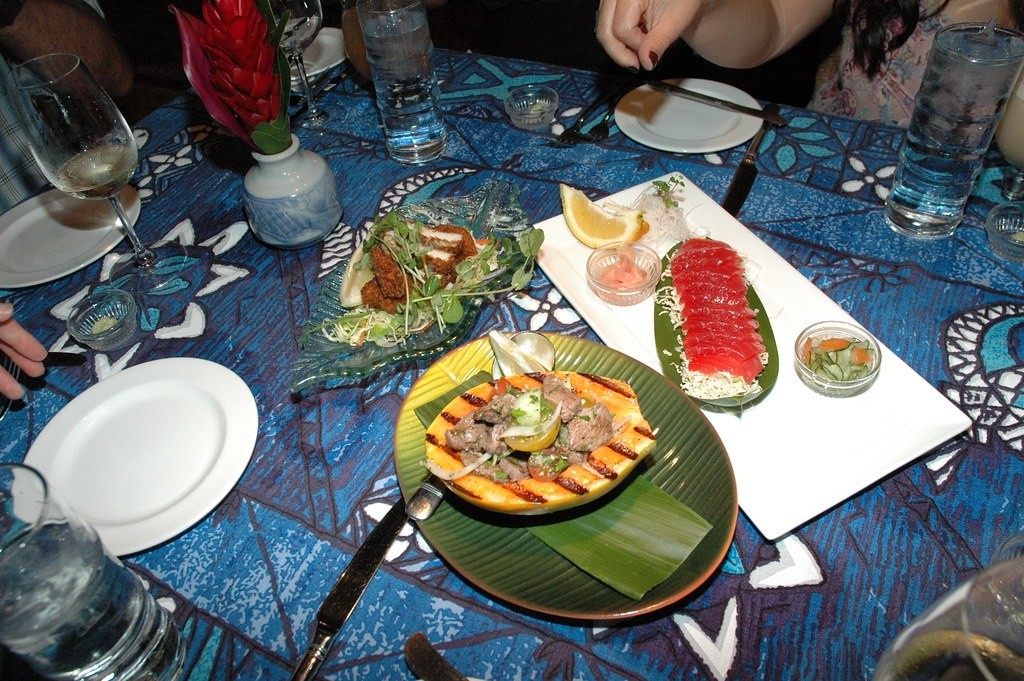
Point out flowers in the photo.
[169,0,292,155]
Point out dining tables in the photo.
[0,48,1024,681]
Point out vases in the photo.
[244,133,342,249]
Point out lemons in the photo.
[559,183,649,248]
[489,329,548,376]
[340,242,375,307]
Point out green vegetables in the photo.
[451,385,599,481]
[303,208,545,340]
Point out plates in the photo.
[281,25,349,84]
[0,181,142,291]
[613,78,766,154]
[393,328,739,619]
[522,170,975,540]
[14,357,261,559]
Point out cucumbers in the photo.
[809,338,874,382]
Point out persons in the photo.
[590,0,1024,169]
[1,1,136,400]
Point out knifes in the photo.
[644,78,788,127]
[287,489,413,681]
[721,102,782,217]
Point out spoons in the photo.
[405,330,557,522]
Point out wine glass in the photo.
[266,0,349,136]
[3,53,173,293]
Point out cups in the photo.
[790,316,883,397]
[505,86,559,130]
[0,462,186,681]
[67,290,137,350]
[585,239,665,307]
[355,1,447,165]
[883,21,1024,239]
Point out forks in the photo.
[540,75,644,147]
